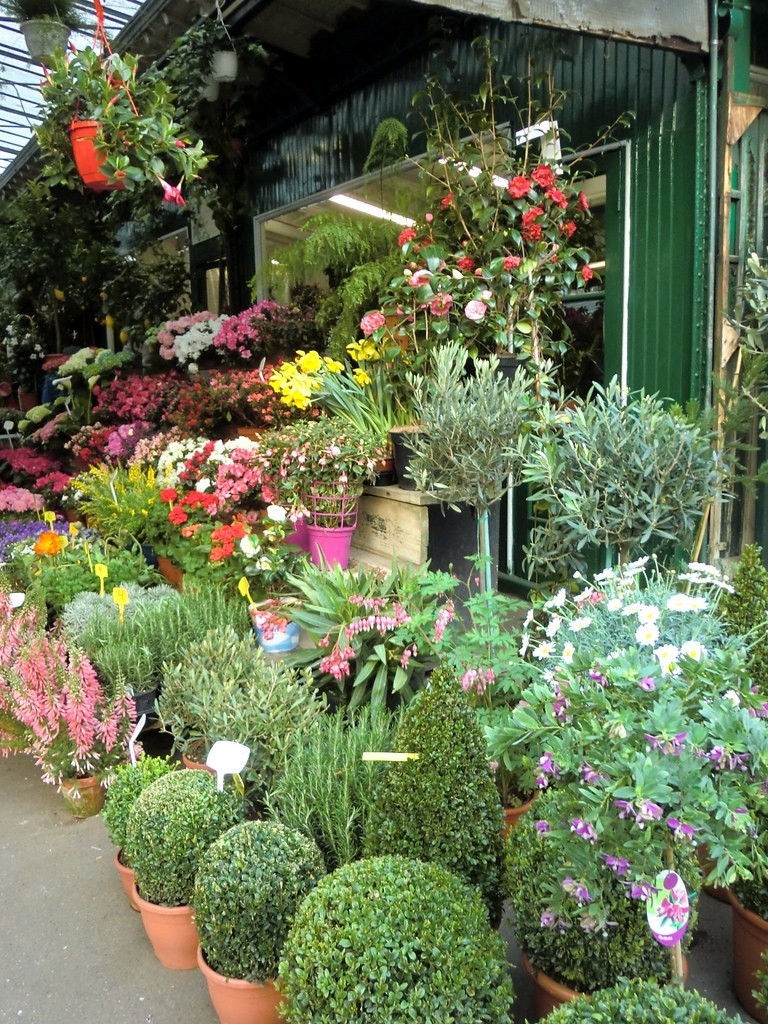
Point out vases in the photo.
[235,427,267,440]
[268,477,309,554]
[57,776,104,814]
[67,122,131,190]
[128,683,155,715]
[158,558,185,585]
[138,544,159,568]
[248,596,302,653]
[16,389,37,410]
[386,429,434,491]
[364,451,397,486]
[305,477,358,572]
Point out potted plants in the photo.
[164,20,275,154]
[0,0,112,65]
[65,585,768,1024]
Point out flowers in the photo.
[0,167,767,953]
[27,38,221,207]
